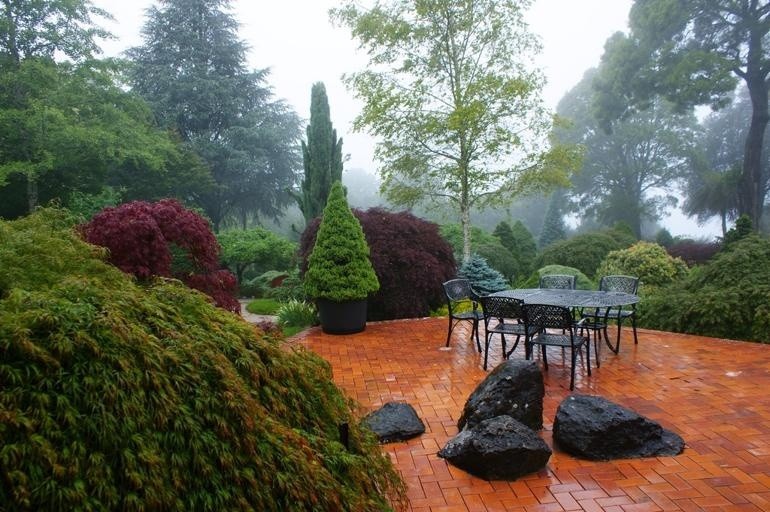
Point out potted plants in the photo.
[299,179,381,335]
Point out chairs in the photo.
[442,271,638,391]
